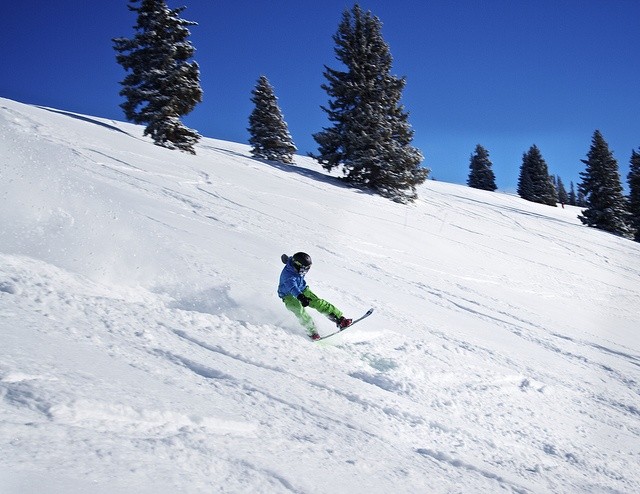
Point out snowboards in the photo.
[320,308,374,340]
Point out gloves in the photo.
[281,254,288,264]
[297,294,312,307]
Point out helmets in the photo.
[291,252,311,270]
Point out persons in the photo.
[277,252,353,341]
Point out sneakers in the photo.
[312,335,320,340]
[339,319,352,328]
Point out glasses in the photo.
[293,259,310,271]
